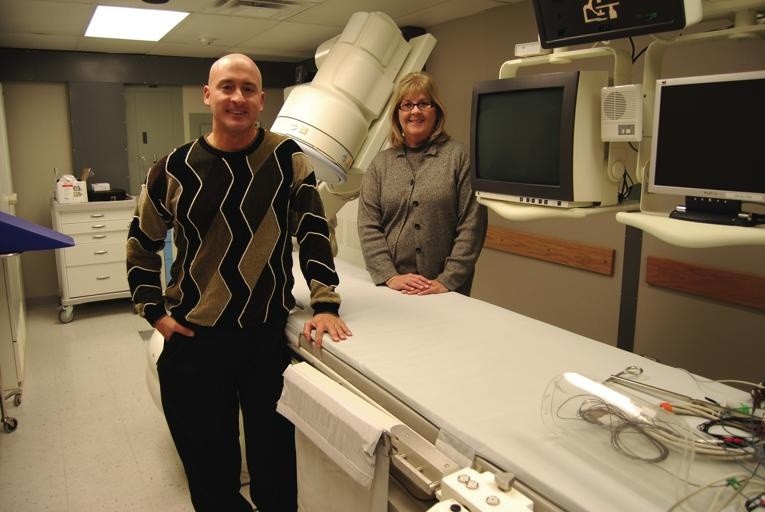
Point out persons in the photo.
[356,72,488,298]
[126,53,353,512]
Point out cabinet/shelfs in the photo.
[0,251,27,392]
[159,230,184,293]
[49,199,160,323]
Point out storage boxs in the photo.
[53,167,91,203]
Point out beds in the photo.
[273,251,764,512]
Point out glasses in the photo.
[398,102,436,111]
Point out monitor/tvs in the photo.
[530,1,702,49]
[648,70,764,227]
[470,69,619,207]
